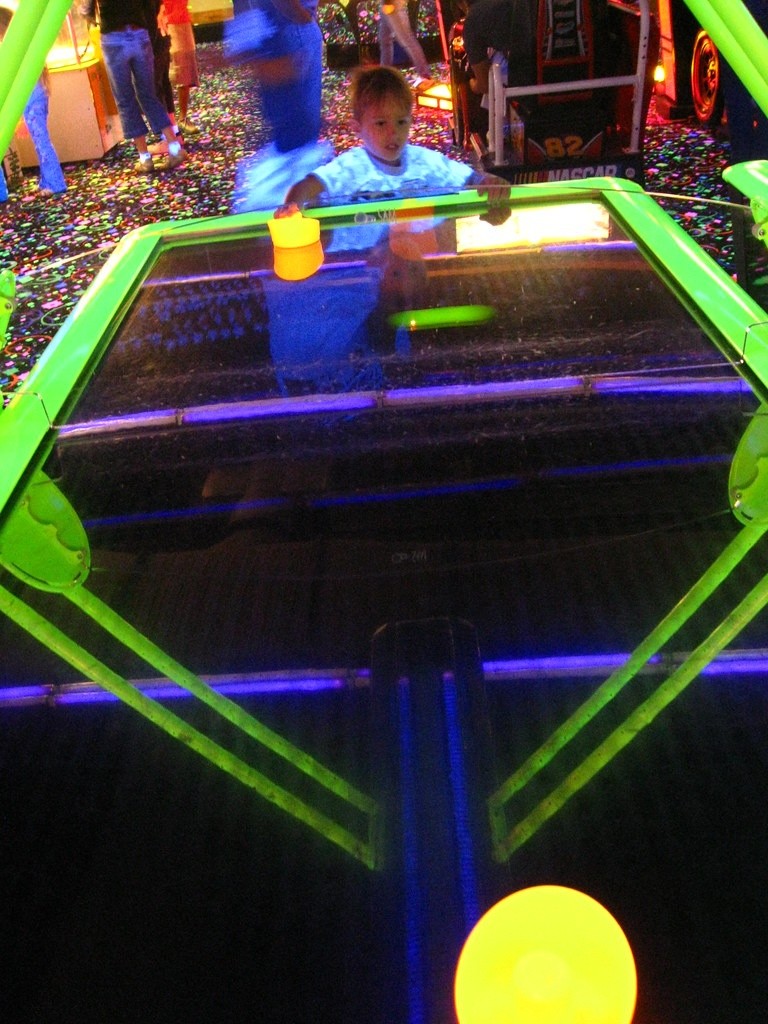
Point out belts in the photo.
[110,24,143,32]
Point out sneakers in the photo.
[162,148,188,169]
[133,157,155,174]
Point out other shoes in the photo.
[146,136,184,153]
[34,188,56,197]
[0,199,13,210]
[177,117,199,134]
[417,78,434,91]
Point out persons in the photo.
[274,68,511,219]
[448,0,611,147]
[0,6,67,200]
[379,0,432,80]
[224,0,324,154]
[75,0,200,173]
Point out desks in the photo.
[0,176,768,1024]
